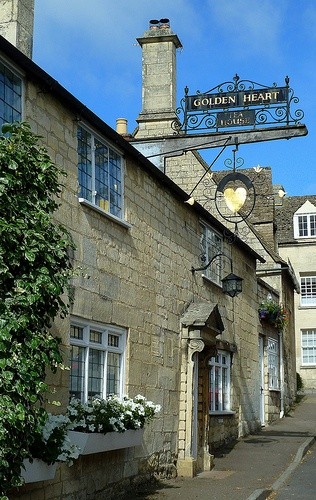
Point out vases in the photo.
[68,428,145,456]
[21,457,57,482]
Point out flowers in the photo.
[65,392,162,434]
[43,412,84,468]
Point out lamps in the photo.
[189,253,244,299]
